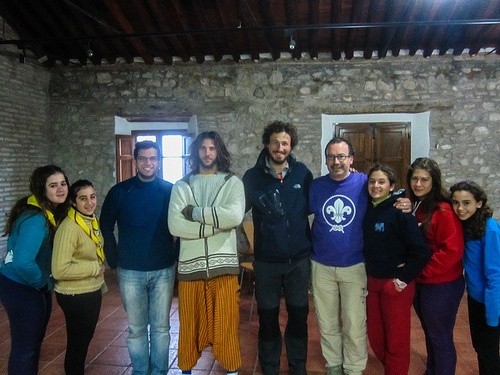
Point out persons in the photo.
[309,138,411,375]
[168,131,245,375]
[242,122,312,375]
[51,180,107,375]
[450,180,500,375]
[0,166,69,375]
[349,165,417,375]
[99,141,177,375]
[406,158,465,375]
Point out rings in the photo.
[403,203,407,206]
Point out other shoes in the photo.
[327,366,342,375]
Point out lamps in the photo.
[290,34,296,49]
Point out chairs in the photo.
[238,221,255,322]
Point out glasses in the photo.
[327,153,348,160]
